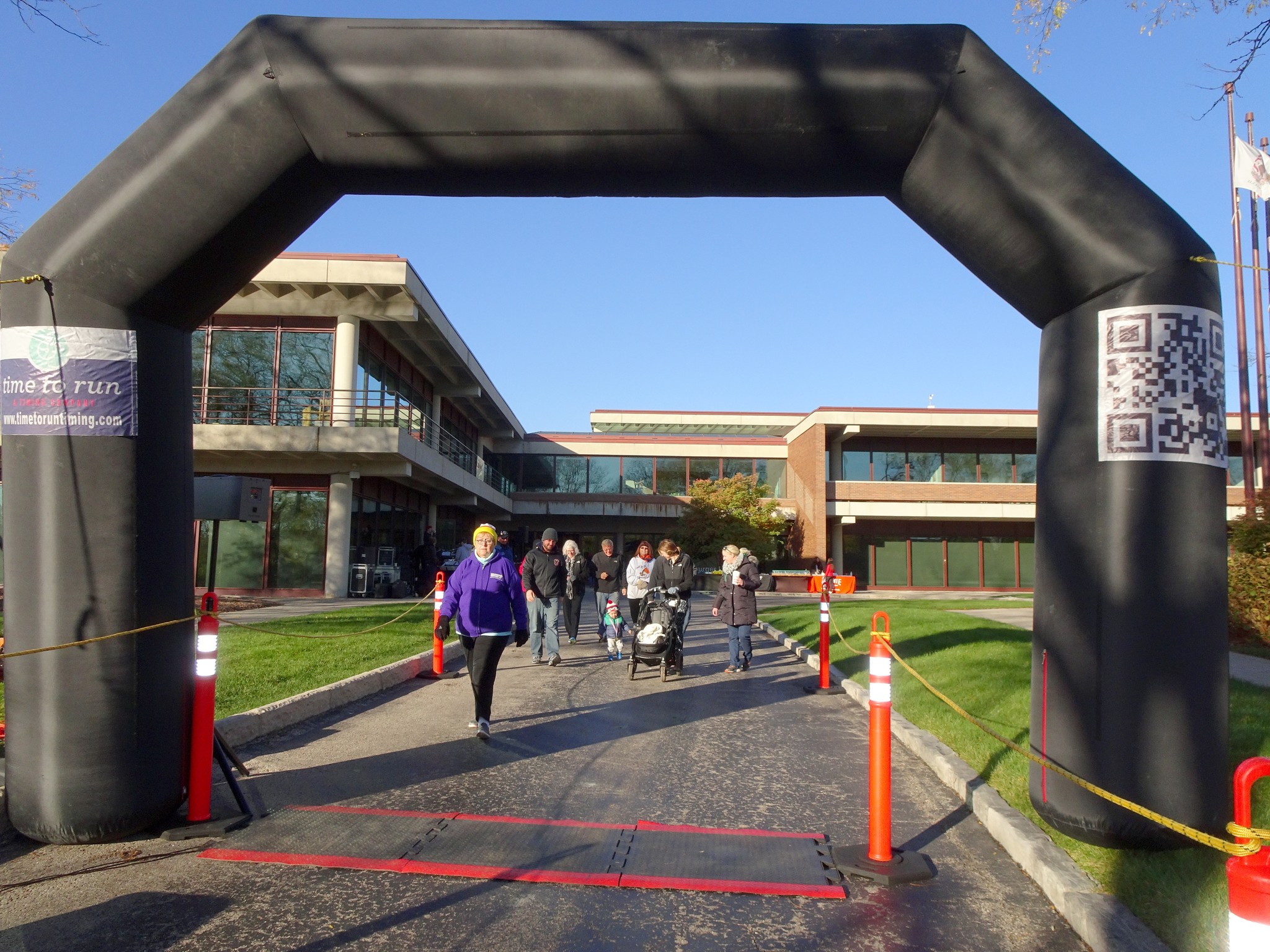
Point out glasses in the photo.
[475,540,495,545]
[566,549,575,552]
[723,545,733,554]
[640,547,649,550]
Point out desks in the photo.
[691,574,857,594]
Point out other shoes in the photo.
[829,591,832,594]
[599,637,607,643]
[568,637,576,645]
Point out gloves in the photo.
[515,629,528,648]
[649,586,662,592]
[434,615,450,642]
[668,586,679,594]
[637,581,648,590]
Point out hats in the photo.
[542,528,558,543]
[500,531,509,539]
[606,600,620,613]
[473,523,498,547]
[429,536,436,543]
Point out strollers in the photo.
[627,587,684,683]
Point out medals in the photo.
[615,637,618,641]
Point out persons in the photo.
[519,528,568,667]
[648,539,697,670]
[712,545,762,673]
[423,525,440,565]
[811,556,823,574]
[626,540,656,628]
[435,523,529,739]
[495,529,513,562]
[454,538,473,565]
[563,539,590,644]
[824,558,835,593]
[597,600,632,661]
[590,539,628,642]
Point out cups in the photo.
[394,563,397,567]
[732,570,741,585]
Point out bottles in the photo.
[850,572,852,575]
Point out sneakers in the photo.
[616,651,622,660]
[532,655,542,664]
[725,664,741,673]
[608,654,613,661]
[742,657,751,671]
[548,654,561,666]
[468,716,480,728]
[477,723,492,738]
